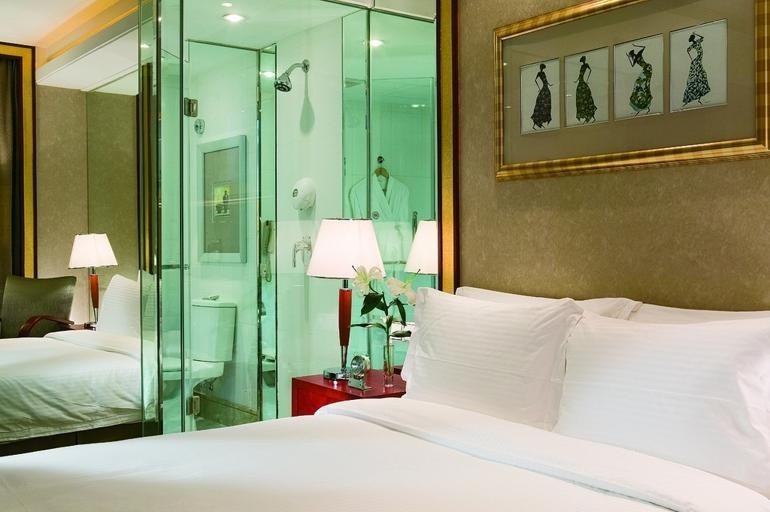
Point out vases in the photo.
[382,344,395,387]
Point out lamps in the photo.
[68,233,118,328]
[307,220,386,380]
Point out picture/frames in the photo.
[493,1,769,182]
[197,135,248,263]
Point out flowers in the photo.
[349,264,421,376]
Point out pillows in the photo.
[92,274,142,361]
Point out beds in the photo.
[0,273,190,444]
[0,282,770,510]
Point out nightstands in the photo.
[293,369,404,416]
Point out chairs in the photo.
[0,276,77,338]
